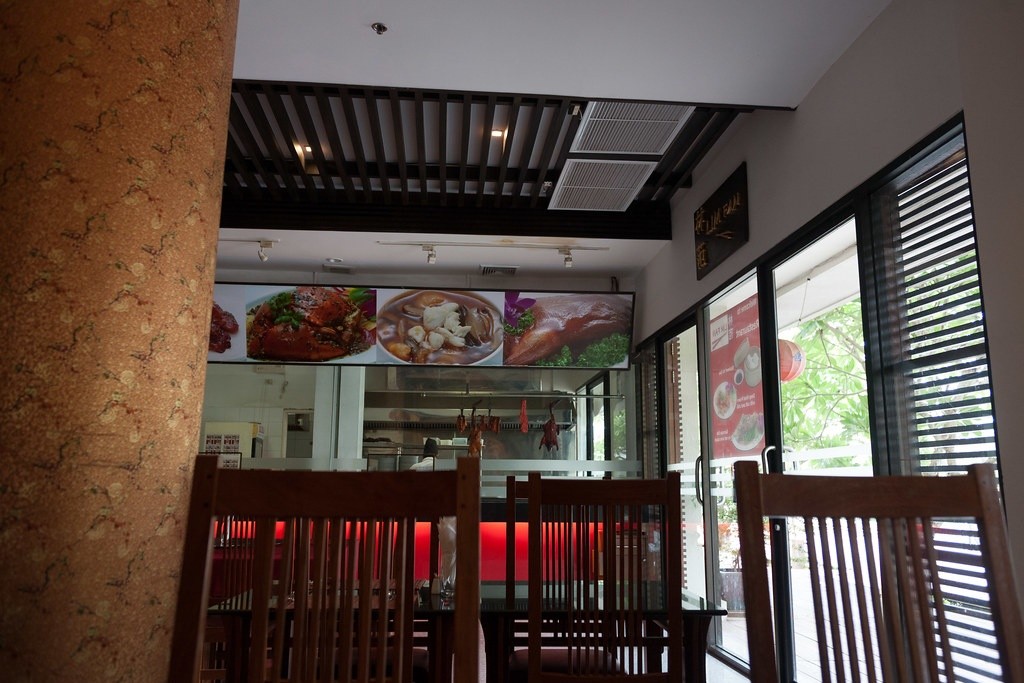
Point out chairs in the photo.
[502,469,683,683]
[168,454,481,683]
[734,460,1024,683]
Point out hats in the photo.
[423,438,438,456]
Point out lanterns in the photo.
[779,339,805,382]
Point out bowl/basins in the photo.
[375,289,503,366]
[423,436,484,446]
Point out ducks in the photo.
[502,291,632,366]
[467,415,484,459]
[538,398,560,452]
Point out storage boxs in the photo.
[440,440,452,445]
[452,438,467,446]
[422,437,440,446]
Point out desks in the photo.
[207,580,728,683]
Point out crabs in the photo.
[248,287,361,360]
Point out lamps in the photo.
[258,242,276,263]
[421,244,437,264]
[557,249,573,267]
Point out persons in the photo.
[409,438,439,472]
